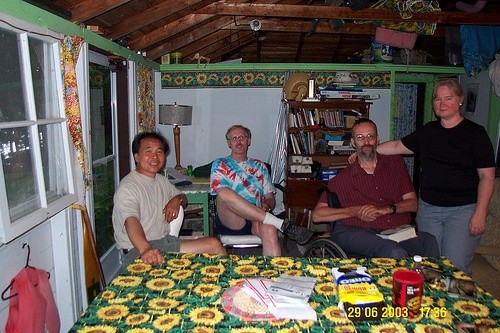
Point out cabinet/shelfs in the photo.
[283,101,373,220]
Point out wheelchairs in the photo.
[302,185,347,259]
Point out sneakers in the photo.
[281,219,313,244]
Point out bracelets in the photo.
[388,203,397,214]
[177,196,184,205]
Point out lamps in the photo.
[251,19,261,32]
[158,102,192,174]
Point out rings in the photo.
[172,213,176,216]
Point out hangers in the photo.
[1,244,50,301]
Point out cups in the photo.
[162,169,169,179]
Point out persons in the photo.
[311,119,440,260]
[112,132,228,264]
[349,78,496,277]
[209,124,314,256]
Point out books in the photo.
[318,82,380,100]
[289,129,315,155]
[376,223,418,243]
[288,107,345,128]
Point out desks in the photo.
[175,184,214,238]
[66,250,500,333]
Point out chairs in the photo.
[218,234,263,254]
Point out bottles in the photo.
[412,255,422,273]
[187,163,193,176]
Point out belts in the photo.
[123,249,128,254]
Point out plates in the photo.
[221,280,276,321]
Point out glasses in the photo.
[352,133,378,142]
[228,135,252,142]
